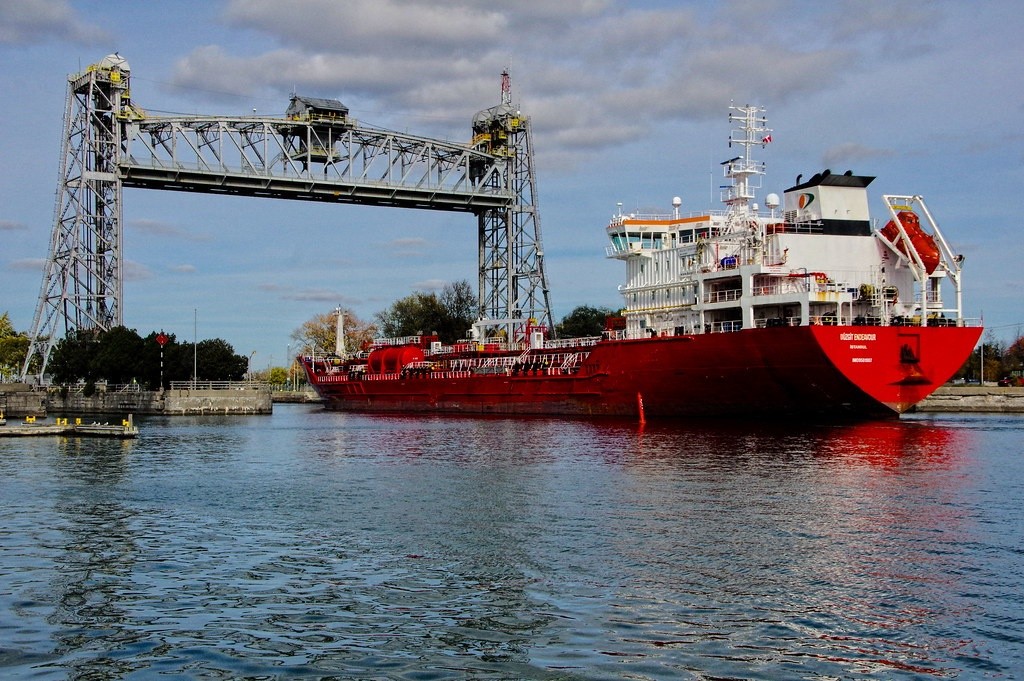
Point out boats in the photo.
[296,99,985,421]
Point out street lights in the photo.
[250,351,256,387]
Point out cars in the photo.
[998,375,1024,387]
